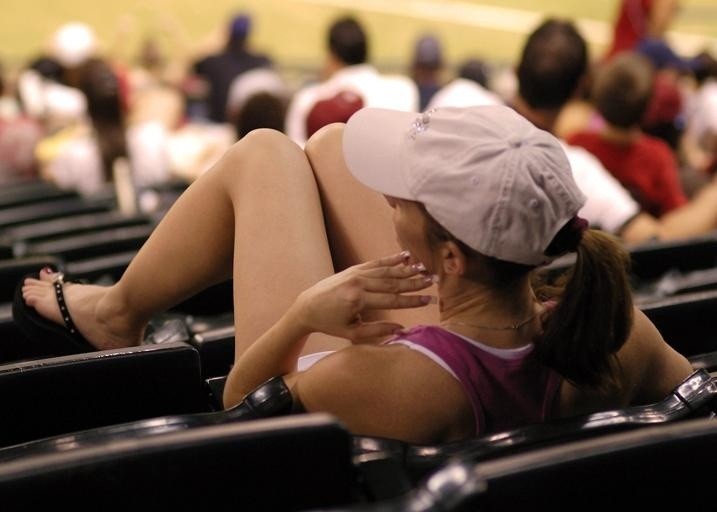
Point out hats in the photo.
[342,103,588,270]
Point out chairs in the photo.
[0,168,717,511]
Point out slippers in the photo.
[11,263,147,387]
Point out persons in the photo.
[19,104,690,447]
[1,11,717,247]
